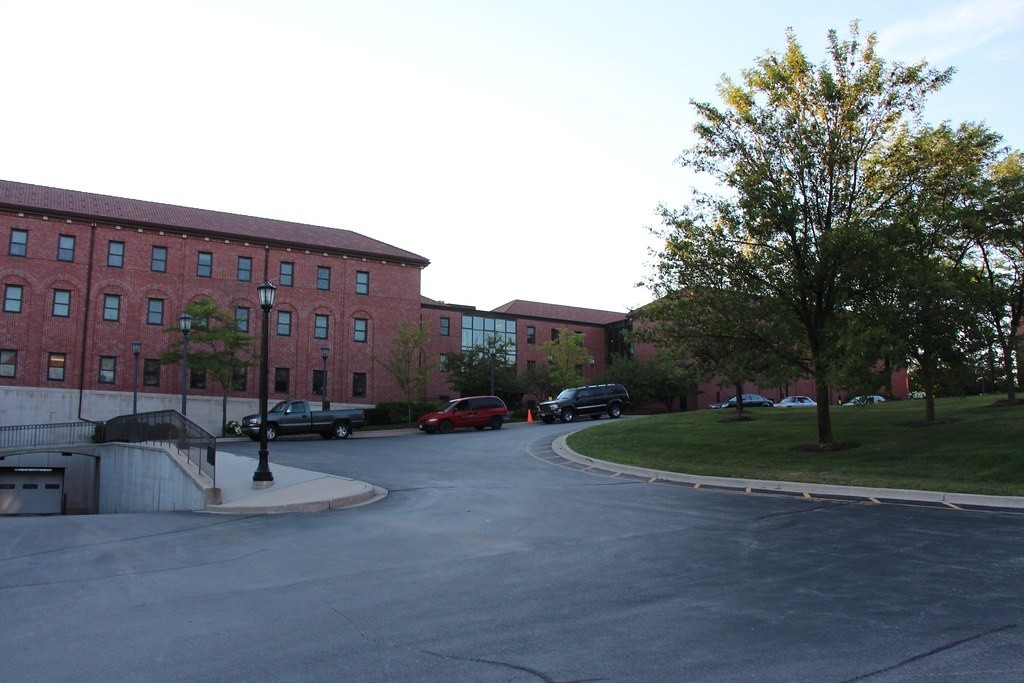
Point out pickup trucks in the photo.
[416,395,508,434]
[539,383,631,423]
[240,398,366,442]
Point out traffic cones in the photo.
[527,409,533,423]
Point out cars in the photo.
[708,392,773,409]
[775,396,817,409]
[841,395,886,407]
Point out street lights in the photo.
[178,312,191,450]
[490,345,495,396]
[320,346,330,410]
[252,280,279,492]
[130,340,142,443]
